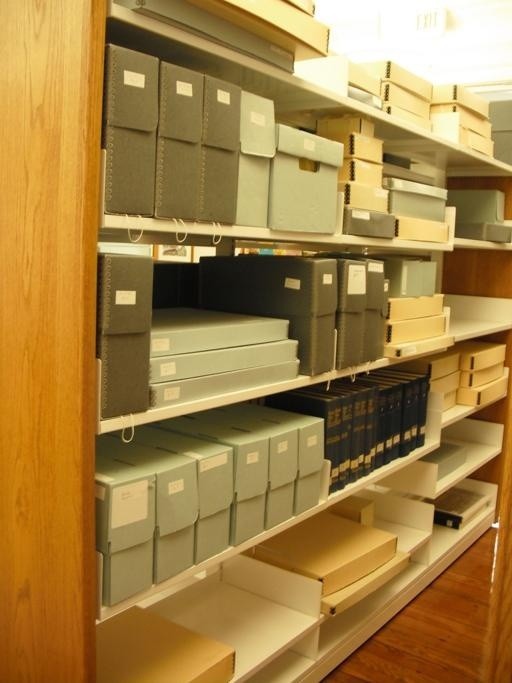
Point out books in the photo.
[413,486,493,528]
[276,369,430,494]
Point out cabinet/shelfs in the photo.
[1,0,511,683]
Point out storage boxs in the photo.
[95,0,512,603]
